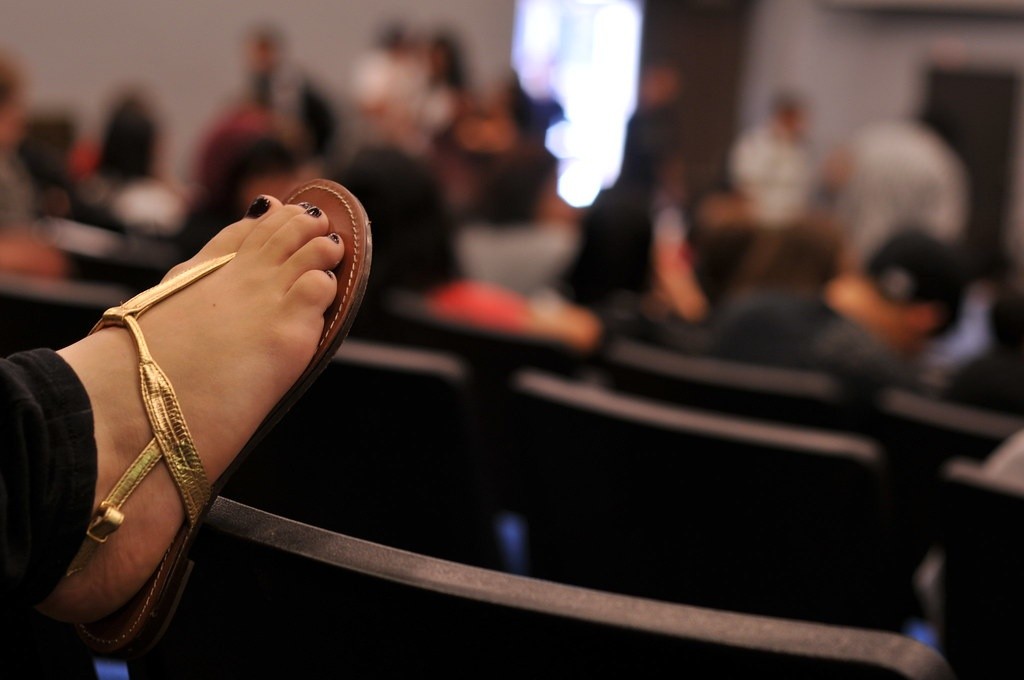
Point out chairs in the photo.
[2,213,1024,679]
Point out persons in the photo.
[0,28,1024,494]
[0,177,373,662]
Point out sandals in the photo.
[61,179,372,657]
